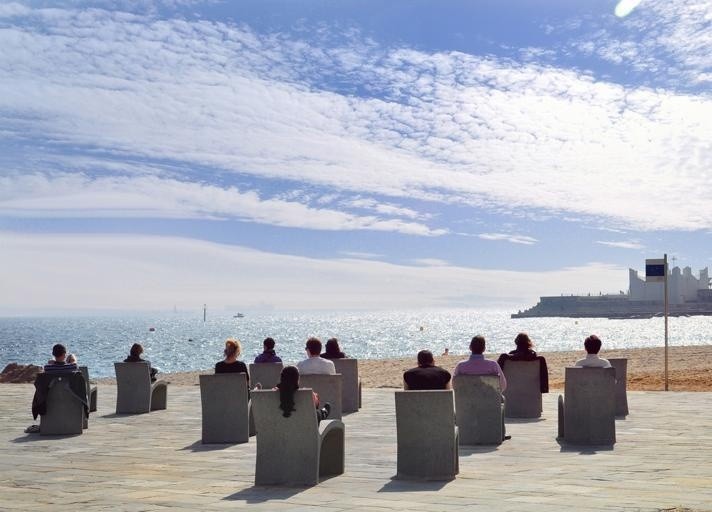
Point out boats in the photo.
[233,312,243,318]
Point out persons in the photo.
[123,342,158,382]
[402,349,452,390]
[320,337,346,358]
[214,337,263,402]
[271,365,331,426]
[508,332,537,357]
[442,348,449,355]
[296,336,336,374]
[451,335,512,441]
[48,343,67,364]
[254,337,282,363]
[66,353,77,363]
[575,334,612,367]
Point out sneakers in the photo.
[322,403,330,419]
[24,425,39,433]
